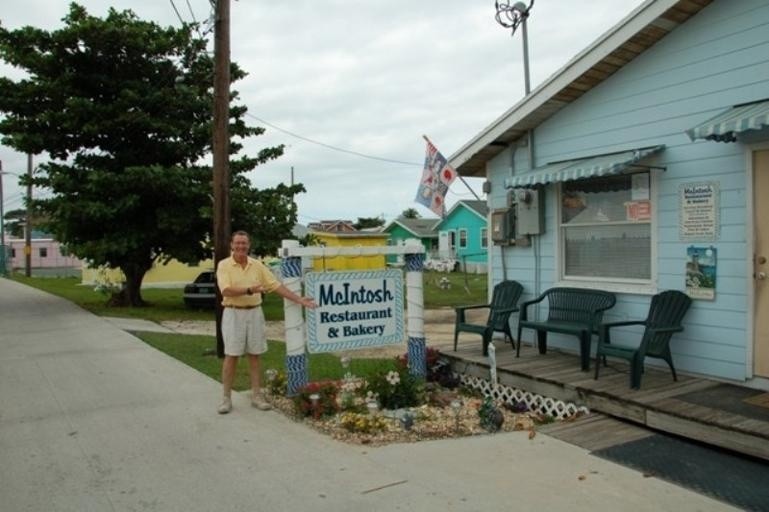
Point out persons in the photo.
[215,230,320,417]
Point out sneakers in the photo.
[216,395,233,414]
[250,392,273,411]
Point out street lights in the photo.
[1,171,33,277]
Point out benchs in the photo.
[517,288,616,371]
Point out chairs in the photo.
[454,280,523,357]
[594,291,693,391]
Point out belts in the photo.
[224,304,261,310]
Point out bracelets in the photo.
[247,287,254,296]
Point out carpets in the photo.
[669,383,769,421]
[589,433,769,512]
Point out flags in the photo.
[413,140,459,221]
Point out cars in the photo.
[183,271,216,307]
